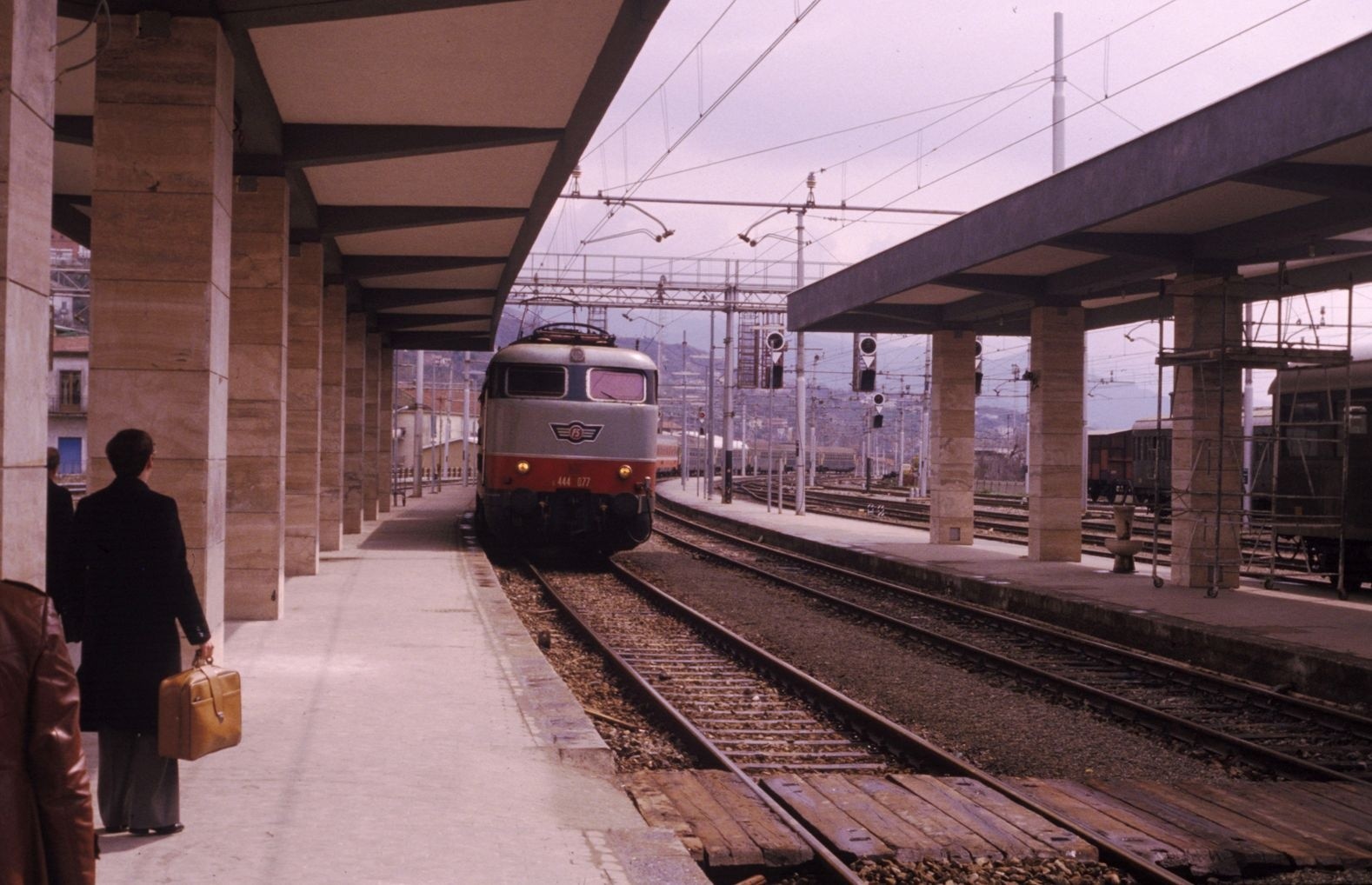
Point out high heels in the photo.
[106,825,126,832]
[129,824,184,835]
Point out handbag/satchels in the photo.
[159,651,242,761]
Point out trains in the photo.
[1086,358,1372,583]
[474,297,856,554]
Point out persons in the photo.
[65,428,214,836]
[46,447,73,578]
[0,578,96,885]
